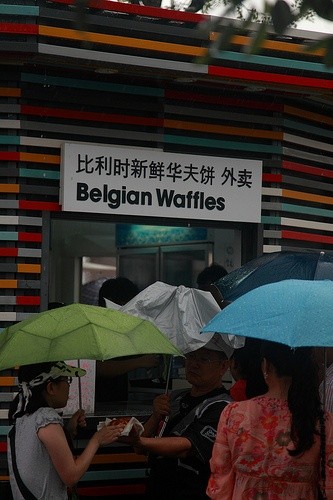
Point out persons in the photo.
[313,346,333,412]
[228,337,269,404]
[206,339,333,500]
[124,332,235,500]
[95,278,159,402]
[197,264,230,310]
[6,361,125,500]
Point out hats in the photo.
[17,360,87,393]
[201,332,236,361]
